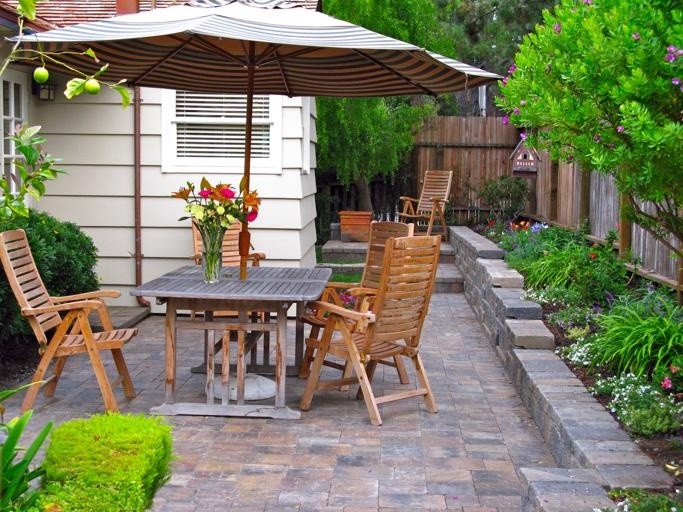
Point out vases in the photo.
[195,223,228,284]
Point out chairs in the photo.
[299,220,414,391]
[396,170,453,243]
[301,234,442,426]
[0,228,139,415]
[192,213,266,323]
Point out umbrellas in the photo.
[5,1,505,281]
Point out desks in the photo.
[128,265,332,421]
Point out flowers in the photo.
[169,175,262,280]
